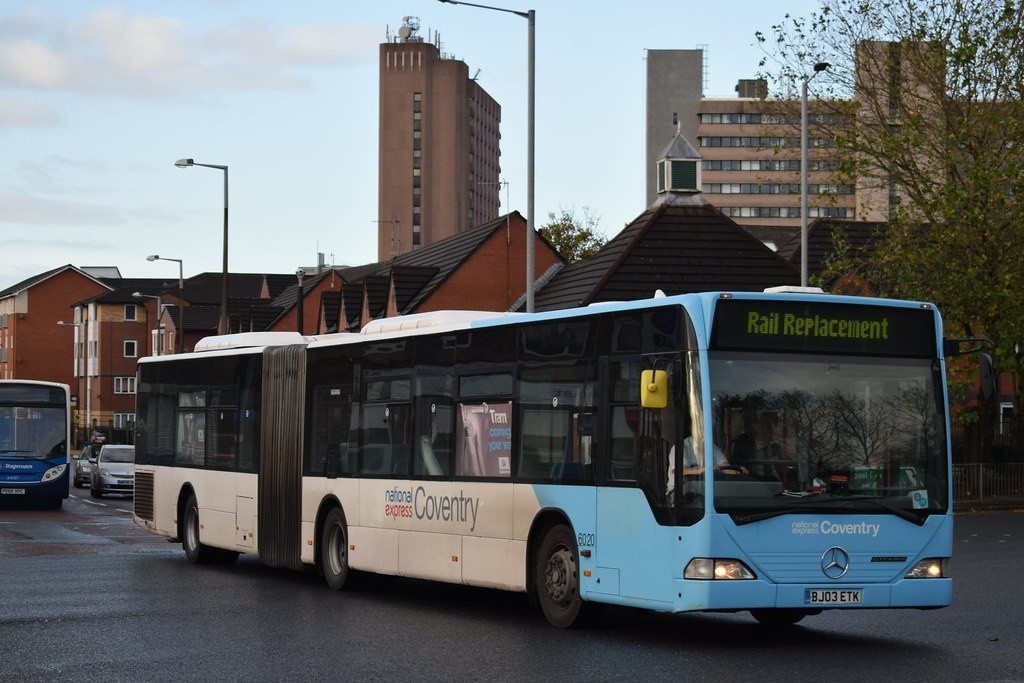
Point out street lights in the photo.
[438,0,536,312]
[173,158,228,334]
[146,255,183,354]
[57,320,79,449]
[800,62,831,288]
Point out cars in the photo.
[88,444,135,499]
[72,444,102,487]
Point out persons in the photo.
[175,440,194,465]
[665,420,742,508]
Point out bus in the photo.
[0,379,70,509]
[131,285,955,628]
